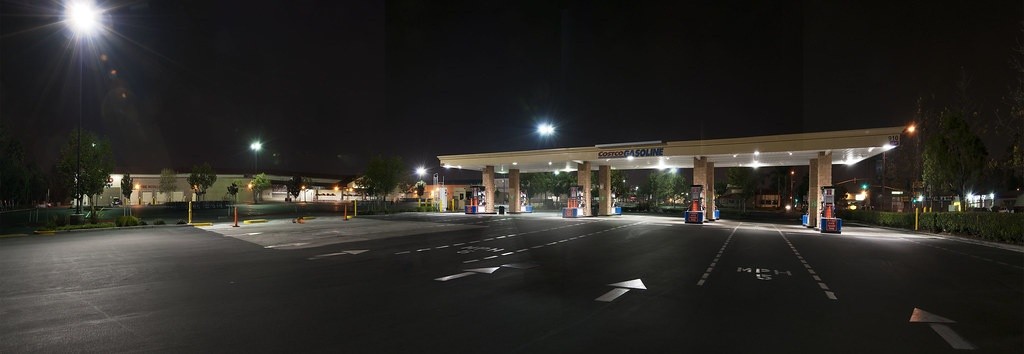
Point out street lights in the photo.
[251,141,262,179]
[536,122,556,204]
[415,167,427,202]
[61,0,97,213]
[908,124,917,212]
[790,171,795,217]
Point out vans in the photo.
[757,198,778,209]
